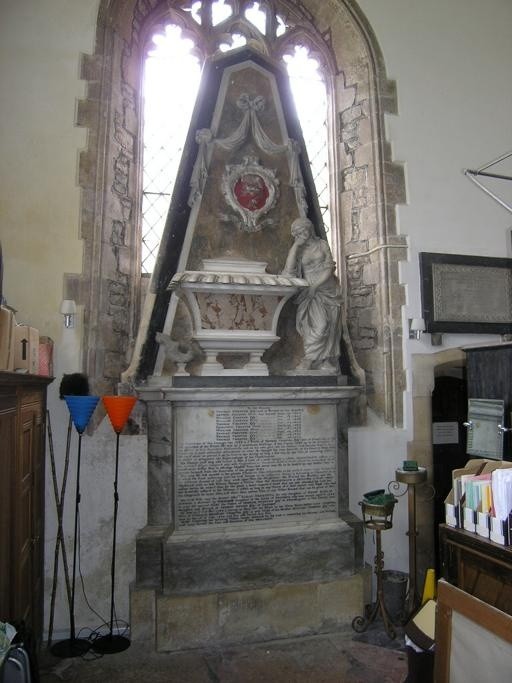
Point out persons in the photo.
[279,217,346,375]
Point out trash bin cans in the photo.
[405,599,437,683]
[377,570,408,622]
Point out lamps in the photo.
[52,392,142,659]
[59,297,79,330]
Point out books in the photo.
[452,466,511,524]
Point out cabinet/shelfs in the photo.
[1,370,56,682]
